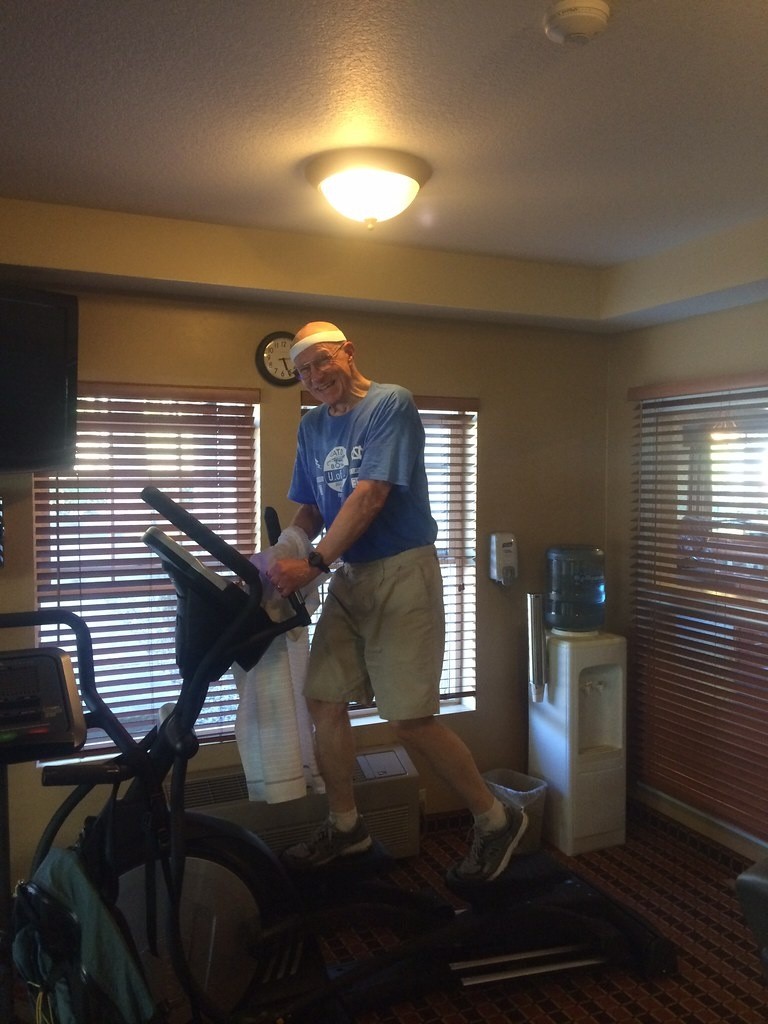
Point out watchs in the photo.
[308,551,330,573]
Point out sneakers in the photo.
[453,802,529,886]
[285,816,373,867]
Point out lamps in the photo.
[304,148,435,230]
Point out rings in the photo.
[277,585,283,592]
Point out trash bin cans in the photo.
[480,767,548,854]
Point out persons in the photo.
[268,322,527,887]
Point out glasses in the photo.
[292,342,345,380]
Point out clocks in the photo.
[254,330,300,388]
